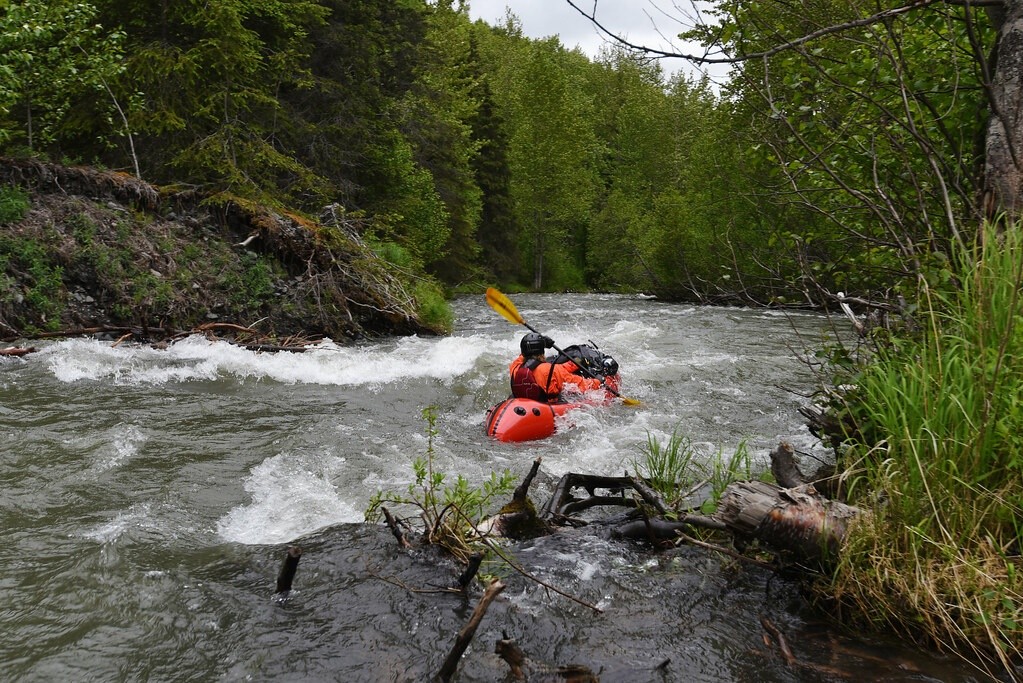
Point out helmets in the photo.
[521,333,545,356]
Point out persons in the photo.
[509,332,606,402]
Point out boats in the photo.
[485,356,620,443]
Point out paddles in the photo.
[486,288,642,406]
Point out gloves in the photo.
[595,374,606,384]
[542,335,555,349]
